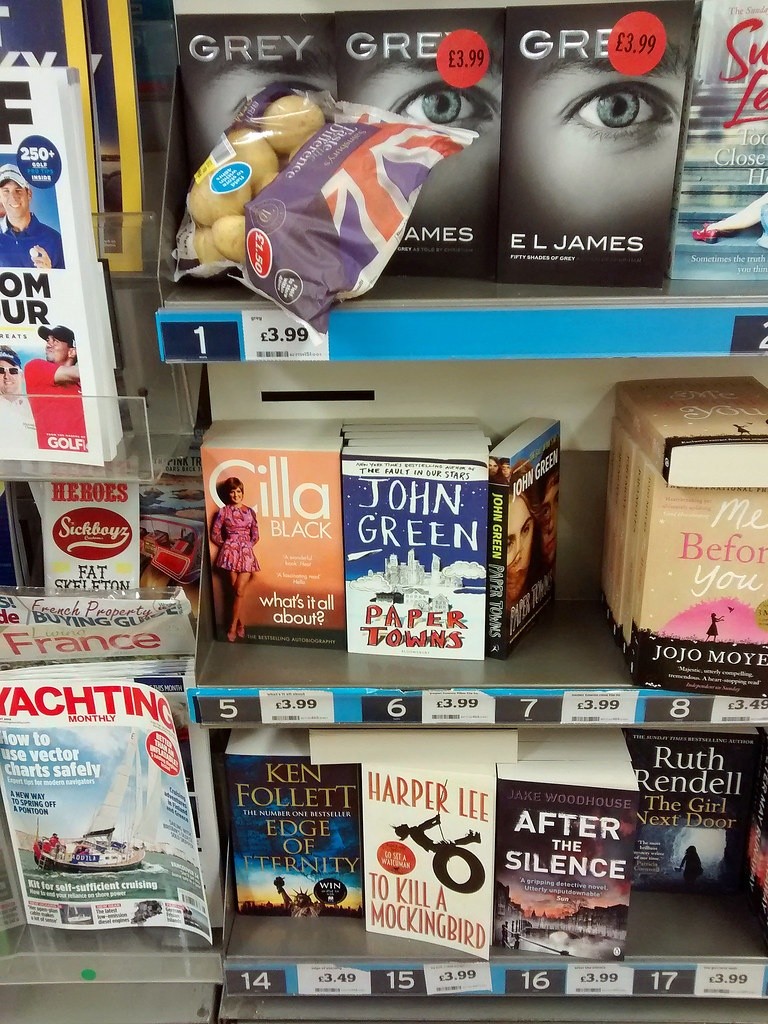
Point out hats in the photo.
[0,345,22,368]
[37,325,76,349]
[0,164,30,190]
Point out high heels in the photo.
[692,223,716,241]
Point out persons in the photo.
[336,8,506,281]
[489,441,559,612]
[210,477,261,642]
[0,164,65,270]
[502,0,697,289]
[0,325,88,451]
[691,192,768,244]
[178,8,338,280]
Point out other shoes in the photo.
[227,632,237,642]
[237,618,245,639]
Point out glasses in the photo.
[0,367,18,375]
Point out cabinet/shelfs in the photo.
[0,0,768,1024]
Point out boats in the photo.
[32,828,147,873]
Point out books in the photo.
[0,68,124,466]
[0,656,226,945]
[599,376,768,699]
[200,416,492,660]
[484,417,560,661]
[0,0,149,273]
[223,724,768,963]
[173,0,768,290]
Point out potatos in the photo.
[188,94,326,266]
